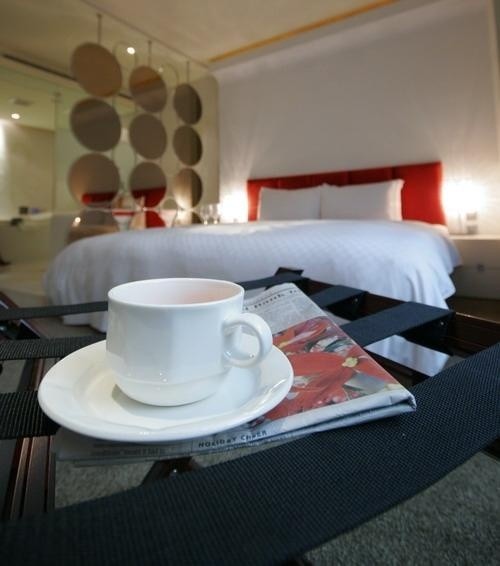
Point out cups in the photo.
[108,278,273,409]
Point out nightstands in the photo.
[450,233,500,300]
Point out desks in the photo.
[0,267,500,566]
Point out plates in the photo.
[35,333,295,444]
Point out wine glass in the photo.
[200,203,222,225]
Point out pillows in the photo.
[254,176,408,223]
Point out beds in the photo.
[45,159,467,334]
